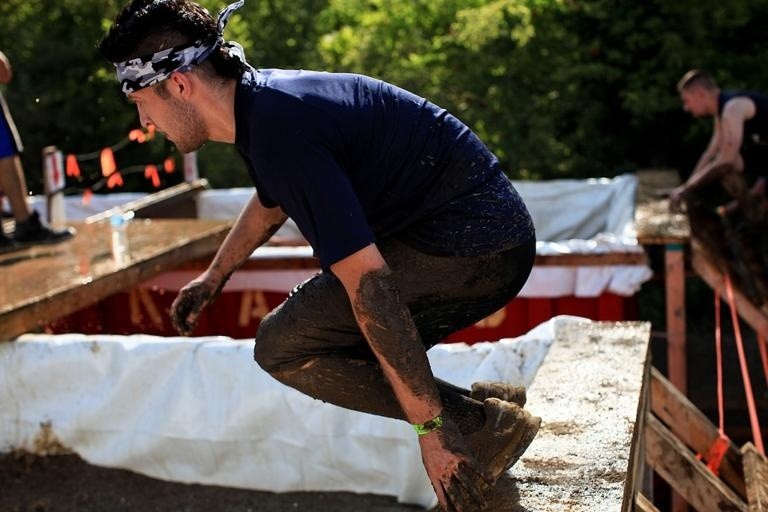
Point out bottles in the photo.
[110,205,130,265]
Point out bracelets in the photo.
[412,409,448,437]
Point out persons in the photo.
[668,65,768,308]
[93,1,545,510]
[0,51,77,253]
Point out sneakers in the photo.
[0,209,77,255]
[435,381,543,512]
